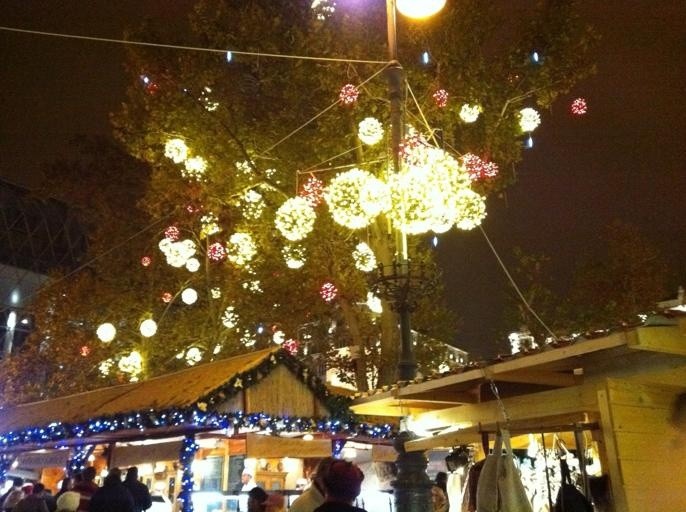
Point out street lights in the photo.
[385,1,448,382]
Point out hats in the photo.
[242,465,255,477]
[323,458,364,496]
[249,487,268,502]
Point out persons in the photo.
[0,466,152,512]
[288,456,337,512]
[312,459,367,512]
[434,471,449,512]
[247,486,268,512]
[238,458,257,512]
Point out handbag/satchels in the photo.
[469,430,532,512]
[555,460,594,511]
